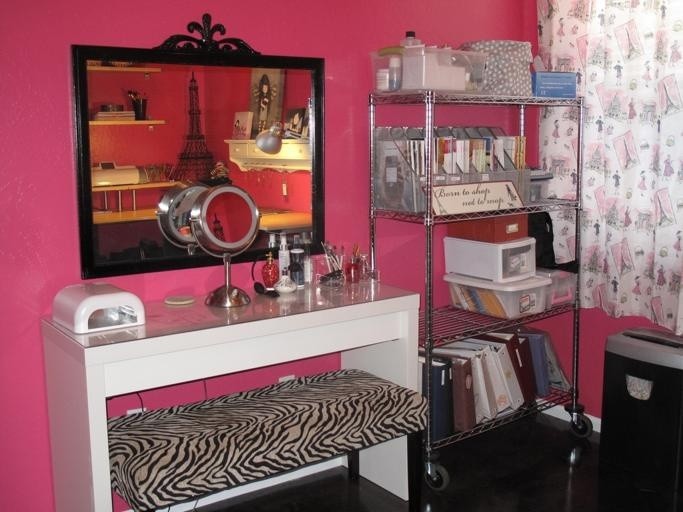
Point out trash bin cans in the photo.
[598,329,683,498]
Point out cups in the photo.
[135,100,146,120]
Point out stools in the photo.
[106,369,431,512]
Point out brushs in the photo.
[398,30,422,57]
[292,230,312,245]
[319,239,346,284]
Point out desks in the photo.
[91,179,312,231]
[38,285,421,512]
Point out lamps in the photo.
[255,120,309,156]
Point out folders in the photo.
[419,331,549,443]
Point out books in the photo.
[417,323,574,443]
[280,106,310,140]
[230,109,255,140]
[372,136,526,212]
[92,110,136,123]
[445,282,542,319]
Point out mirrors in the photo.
[154,181,212,255]
[189,182,262,308]
[71,13,327,280]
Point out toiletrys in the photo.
[278,231,288,246]
[260,241,314,293]
[265,232,277,248]
[357,253,372,280]
[385,55,402,92]
[346,255,360,284]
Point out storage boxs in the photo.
[530,70,578,98]
[401,46,487,95]
[368,47,405,96]
[530,168,554,204]
[442,209,578,320]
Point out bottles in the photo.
[388,56,403,90]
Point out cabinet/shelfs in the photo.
[369,91,593,493]
[87,65,166,126]
